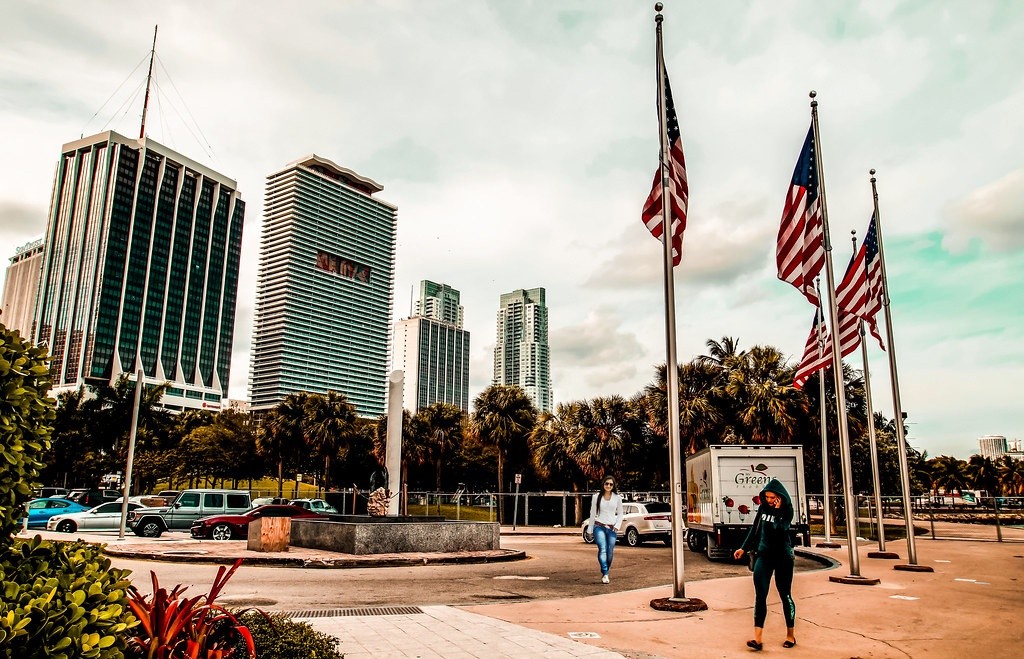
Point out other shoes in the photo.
[601,575,610,584]
[783,638,796,648]
[747,640,763,650]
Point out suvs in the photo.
[126,488,253,538]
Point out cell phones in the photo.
[774,498,779,504]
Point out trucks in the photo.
[684,445,810,562]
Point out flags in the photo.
[641,27,688,267]
[834,209,886,352]
[794,249,864,389]
[776,117,827,307]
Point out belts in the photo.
[595,521,613,529]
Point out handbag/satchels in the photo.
[746,549,757,571]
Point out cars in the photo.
[190,504,330,541]
[158,490,197,506]
[581,500,686,547]
[16,498,93,530]
[28,486,124,506]
[47,501,148,534]
[251,496,289,509]
[288,498,339,515]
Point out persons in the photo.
[587,476,624,583]
[734,479,797,650]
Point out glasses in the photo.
[604,482,614,486]
